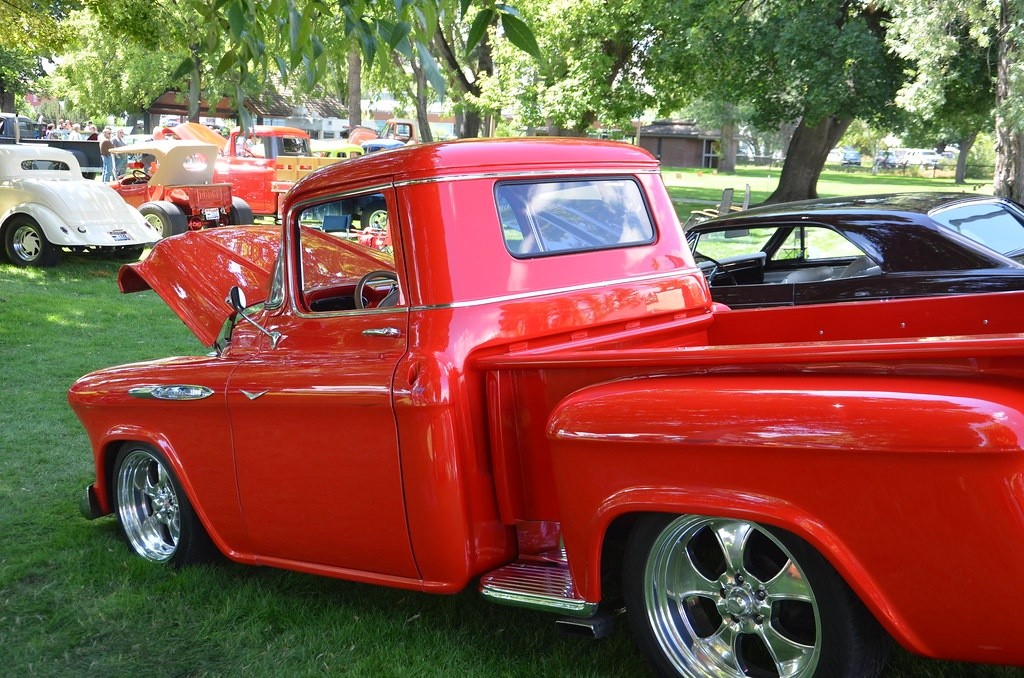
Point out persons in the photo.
[41,118,181,182]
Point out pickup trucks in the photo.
[0,112,102,180]
[350,118,419,149]
[309,138,405,160]
[162,122,345,216]
[68,137,1024,678]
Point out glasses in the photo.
[119,132,124,133]
[106,133,110,134]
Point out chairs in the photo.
[782,266,833,283]
[716,184,750,211]
[838,256,877,279]
[519,198,624,252]
[682,188,734,233]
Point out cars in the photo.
[842,150,861,165]
[0,144,163,269]
[104,141,254,244]
[875,148,954,168]
[527,191,1024,310]
